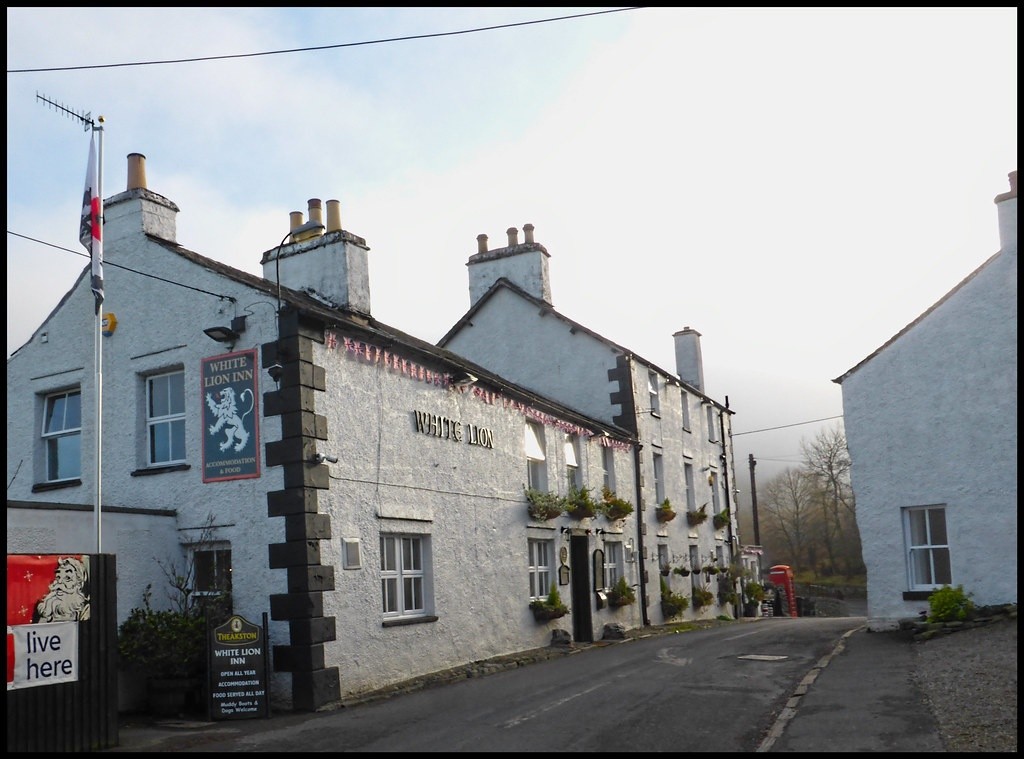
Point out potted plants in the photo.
[607,575,637,608]
[660,564,671,577]
[686,502,709,526]
[659,591,690,620]
[565,485,602,520]
[728,563,749,580]
[529,583,572,623]
[524,486,577,522]
[713,507,732,528]
[703,564,720,575]
[117,509,234,719]
[720,565,728,573]
[743,582,764,607]
[673,565,690,577]
[691,586,715,610]
[599,488,634,520]
[656,497,677,523]
[718,580,740,606]
[692,565,701,576]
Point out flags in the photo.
[78,132,105,316]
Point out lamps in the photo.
[204,325,241,351]
